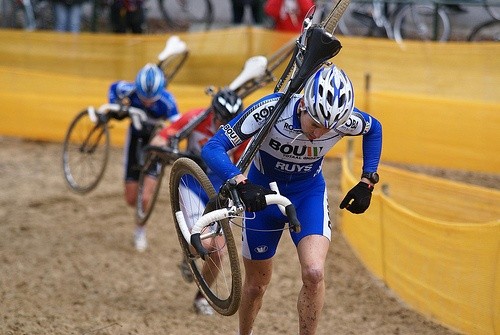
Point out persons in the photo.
[148,87,253,316]
[200,64,382,335]
[0,0,316,35]
[107,62,189,253]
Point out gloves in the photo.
[235,179,277,212]
[340,181,374,214]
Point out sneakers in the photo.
[179,258,194,283]
[134,227,147,252]
[193,296,214,314]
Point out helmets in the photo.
[212,90,243,122]
[304,64,354,130]
[136,62,165,98]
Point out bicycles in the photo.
[62,36,191,196]
[1,0,500,44]
[168,0,354,317]
[134,33,303,226]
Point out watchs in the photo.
[361,172,379,184]
